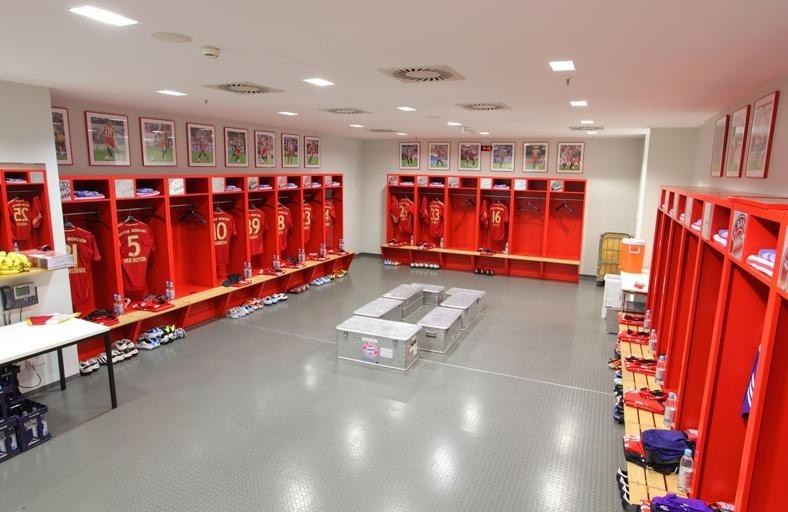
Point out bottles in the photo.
[648,329,657,355]
[504,242,508,256]
[643,309,652,333]
[677,449,692,494]
[409,235,413,246]
[655,356,665,384]
[439,238,443,250]
[663,392,675,427]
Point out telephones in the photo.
[0,282,39,325]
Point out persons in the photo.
[561,145,580,169]
[286,140,294,164]
[259,137,269,163]
[526,146,540,169]
[231,133,244,162]
[159,126,169,162]
[95,120,116,158]
[308,142,317,163]
[196,133,208,162]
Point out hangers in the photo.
[410,192,413,201]
[142,206,166,222]
[494,196,500,204]
[179,204,206,224]
[519,197,540,211]
[278,197,283,208]
[215,201,221,213]
[461,194,476,207]
[482,192,487,200]
[402,192,408,200]
[433,192,438,201]
[15,192,23,200]
[123,209,137,225]
[555,198,575,215]
[63,214,76,231]
[618,183,788,512]
[258,196,274,209]
[286,195,298,206]
[86,210,108,229]
[248,199,255,209]
[225,199,244,214]
[327,192,341,202]
[303,195,306,204]
[307,193,321,204]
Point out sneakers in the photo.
[161,325,175,342]
[410,263,414,268]
[434,264,440,269]
[419,263,424,267]
[621,493,629,511]
[608,359,620,368]
[387,260,391,265]
[310,279,320,286]
[474,268,479,273]
[614,385,623,396]
[486,270,489,274]
[143,327,170,344]
[490,270,494,275]
[394,261,400,266]
[279,293,288,300]
[614,371,622,386]
[138,335,160,348]
[272,296,278,304]
[337,271,344,276]
[391,260,395,266]
[87,359,100,372]
[97,351,119,365]
[261,296,272,306]
[425,263,430,269]
[245,299,258,310]
[334,272,342,278]
[414,262,419,267]
[320,277,328,283]
[178,328,186,338]
[616,468,627,483]
[121,339,138,356]
[340,270,345,274]
[481,269,484,274]
[110,341,132,360]
[235,307,245,317]
[224,308,238,318]
[304,283,309,290]
[618,477,628,490]
[612,401,624,424]
[252,297,263,308]
[326,274,333,280]
[616,391,623,402]
[240,305,249,314]
[326,277,331,282]
[317,278,324,285]
[296,285,305,292]
[289,288,301,294]
[175,329,179,340]
[136,337,154,350]
[430,264,433,268]
[332,274,335,279]
[101,350,124,362]
[79,362,92,376]
[247,305,254,312]
[620,485,630,498]
[274,294,281,301]
[344,270,348,274]
[384,259,388,265]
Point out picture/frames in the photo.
[255,129,276,168]
[725,105,751,177]
[85,110,131,165]
[281,132,300,168]
[458,142,481,171]
[745,91,781,179]
[225,126,249,168]
[711,114,729,176]
[186,122,216,167]
[557,142,583,173]
[140,116,177,167]
[491,142,515,172]
[428,142,450,171]
[304,136,320,168]
[522,140,548,173]
[400,141,421,170]
[51,106,74,166]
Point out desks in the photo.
[0,312,119,409]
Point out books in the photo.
[19,248,74,271]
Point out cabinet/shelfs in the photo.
[381,173,586,283]
[58,172,355,364]
[0,163,73,278]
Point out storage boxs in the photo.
[445,287,487,312]
[411,282,445,306]
[606,306,621,334]
[441,291,480,330]
[418,307,463,353]
[352,297,404,320]
[383,284,424,320]
[620,238,646,274]
[601,274,622,319]
[336,315,422,370]
[11,400,52,451]
[0,387,8,416]
[5,388,24,402]
[0,365,19,387]
[1,417,20,462]
